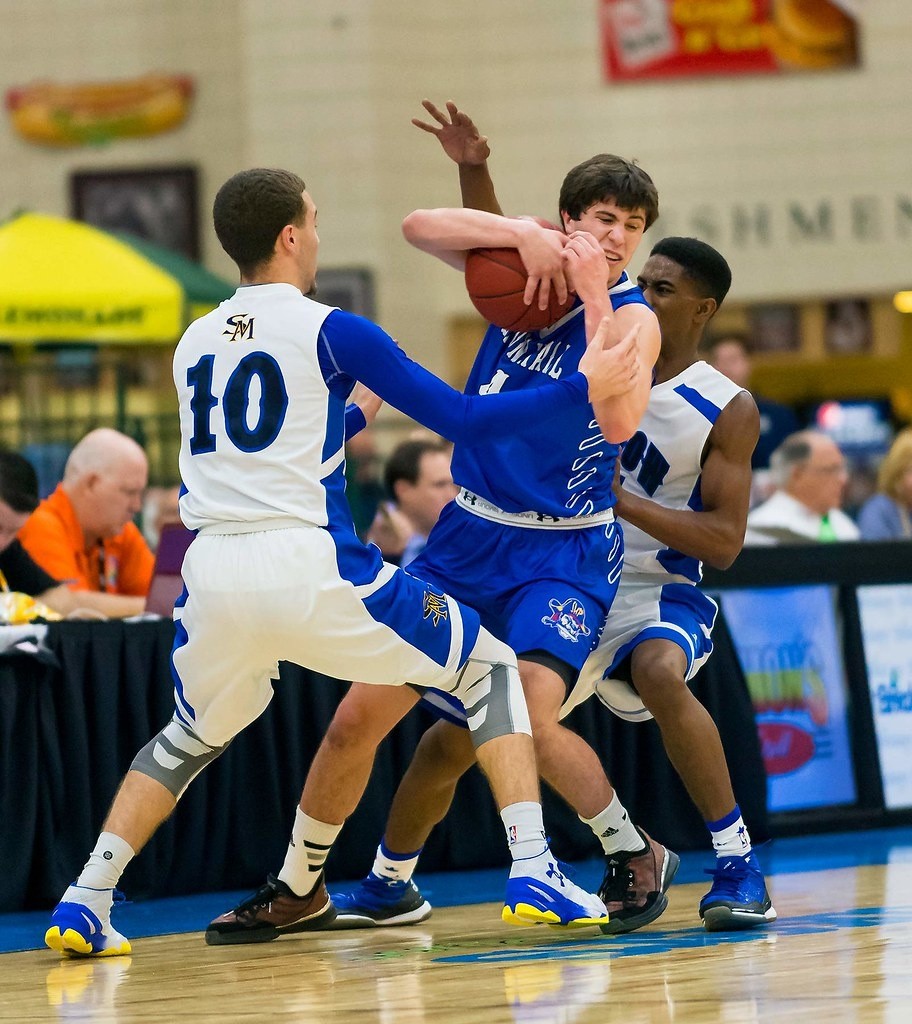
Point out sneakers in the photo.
[698,839,778,931]
[500,847,609,929]
[43,881,132,955]
[206,870,336,945]
[597,824,680,934]
[306,878,433,929]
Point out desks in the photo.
[0,600,732,912]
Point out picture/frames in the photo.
[72,163,202,264]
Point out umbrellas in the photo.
[0,208,238,345]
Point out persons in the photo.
[0,426,195,623]
[364,437,458,566]
[710,332,793,465]
[204,152,677,943]
[741,427,912,547]
[46,170,641,956]
[323,97,777,927]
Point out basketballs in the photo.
[464,214,578,332]
